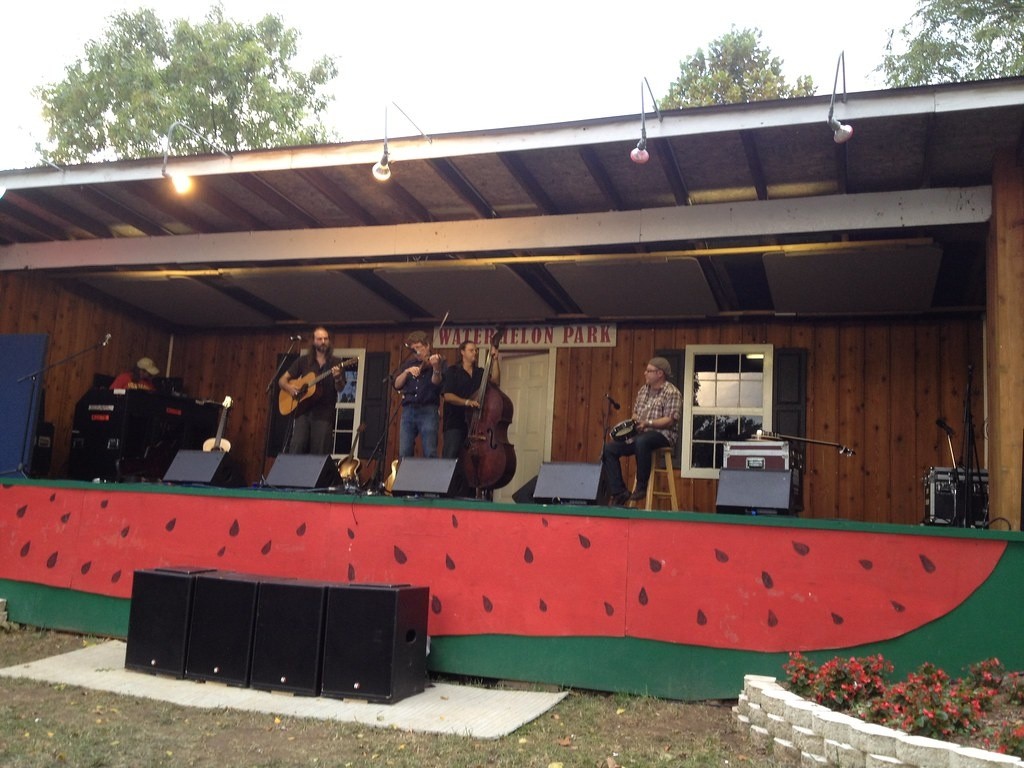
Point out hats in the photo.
[137,357,160,375]
[649,358,671,376]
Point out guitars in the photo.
[337,422,367,490]
[202,395,234,484]
[383,459,398,496]
[278,355,359,419]
[749,428,857,458]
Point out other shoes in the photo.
[614,490,632,506]
[629,489,645,500]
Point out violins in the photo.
[401,354,445,369]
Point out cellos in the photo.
[459,323,517,490]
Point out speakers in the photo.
[263,454,345,494]
[162,450,245,490]
[511,460,601,504]
[716,469,794,517]
[392,457,470,500]
[124,565,430,705]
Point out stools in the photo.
[629,447,678,511]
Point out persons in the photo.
[278,326,347,455]
[603,358,682,507]
[392,331,447,463]
[441,340,500,497]
[109,357,160,393]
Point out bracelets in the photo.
[433,371,440,376]
[405,369,409,374]
[465,400,470,406]
[335,376,342,383]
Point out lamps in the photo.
[372,101,432,181]
[630,77,662,164]
[161,122,232,192]
[827,49,853,143]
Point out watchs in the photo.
[649,419,652,426]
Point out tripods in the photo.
[366,394,407,496]
[0,339,106,480]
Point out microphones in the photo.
[103,334,111,345]
[398,391,417,395]
[605,394,620,409]
[936,419,952,434]
[403,343,421,356]
[290,335,302,340]
